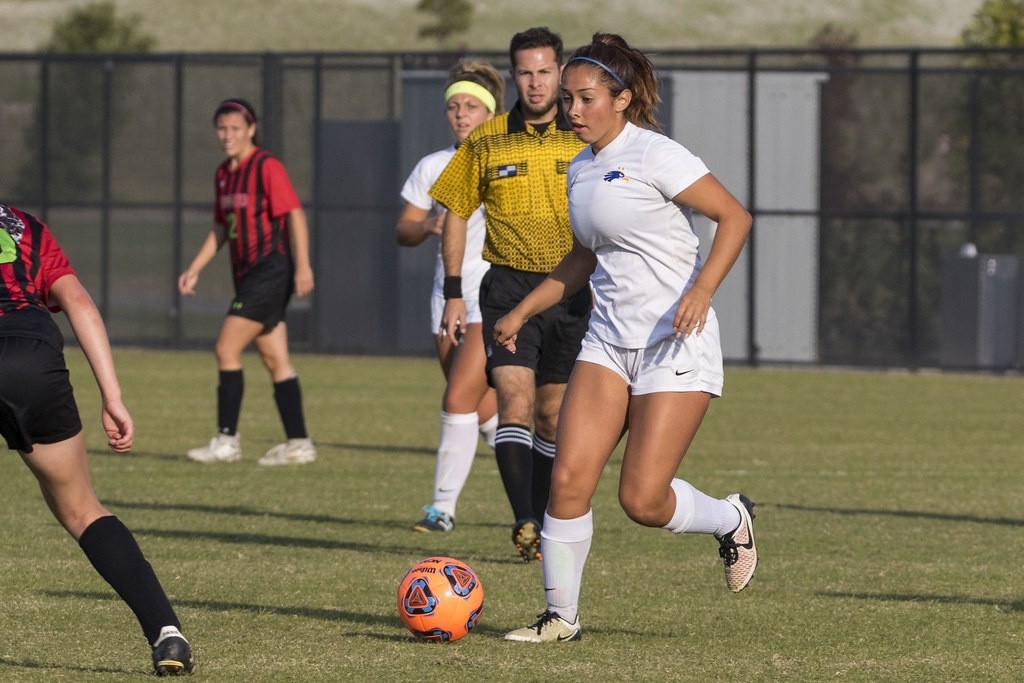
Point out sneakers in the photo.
[412,503,456,534]
[187,431,241,463]
[152,624,196,677]
[511,519,542,562]
[259,436,318,466]
[714,493,759,594]
[504,608,583,643]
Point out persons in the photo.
[177,99,318,466]
[396,25,590,564]
[0,202,195,676]
[493,33,758,643]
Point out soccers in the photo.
[398,556,485,641]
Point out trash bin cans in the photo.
[933,249,1024,371]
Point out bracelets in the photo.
[442,276,464,300]
